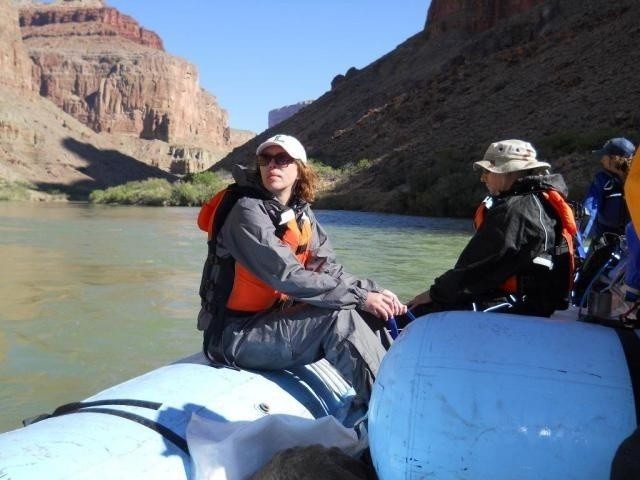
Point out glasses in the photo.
[258,151,296,166]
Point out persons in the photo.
[623,143,640,289]
[196,132,409,410]
[575,136,635,263]
[409,137,578,319]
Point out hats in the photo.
[256,133,308,167]
[473,139,551,174]
[593,137,637,156]
